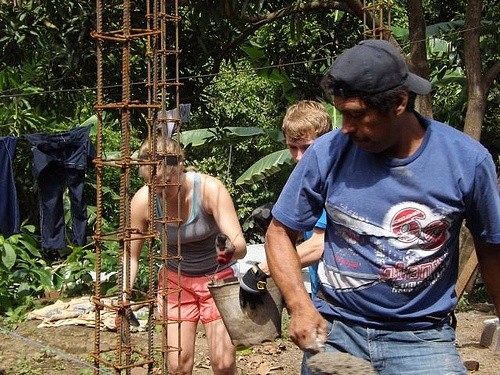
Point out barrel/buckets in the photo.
[207,259,283,346]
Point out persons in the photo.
[117,135,247,375]
[264,39,500,375]
[238,99,337,375]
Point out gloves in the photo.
[215,234,236,267]
[115,309,140,344]
[238,263,272,311]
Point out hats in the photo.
[325,39,431,98]
